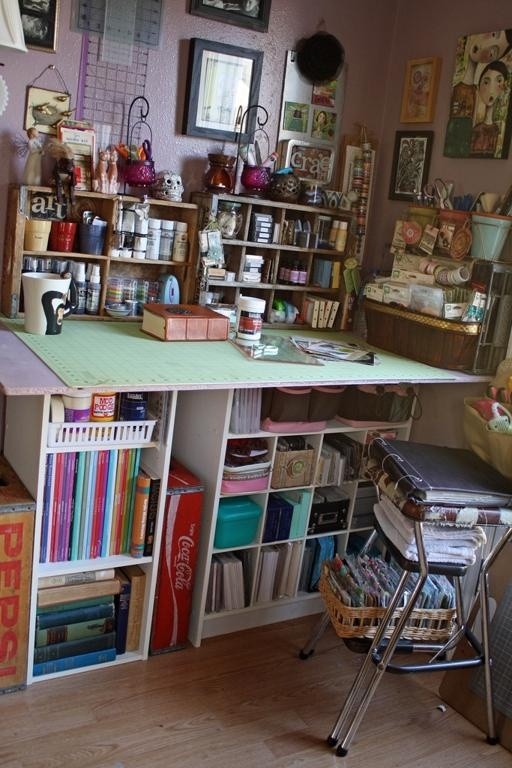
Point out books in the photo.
[140,301,230,344]
[250,211,274,244]
[305,297,339,330]
[34,571,149,678]
[206,557,248,614]
[43,449,158,558]
[368,437,511,503]
[257,545,302,604]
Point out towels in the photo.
[374,493,487,566]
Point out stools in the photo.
[298,482,512,757]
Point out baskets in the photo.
[321,565,457,641]
[271,444,314,489]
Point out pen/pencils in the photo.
[423,196,428,205]
[414,189,418,205]
[419,192,422,196]
[418,196,423,208]
[429,199,433,207]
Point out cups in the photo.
[25,218,53,253]
[51,219,78,252]
[79,222,108,256]
[21,272,80,337]
[320,190,345,209]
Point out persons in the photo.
[10,126,43,188]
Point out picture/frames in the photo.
[17,0,58,54]
[399,56,441,124]
[186,0,272,33]
[443,27,512,160]
[388,130,436,202]
[23,85,71,137]
[181,37,264,144]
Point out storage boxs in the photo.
[0,446,37,693]
[258,385,345,432]
[149,454,206,654]
[332,383,424,427]
[215,495,262,549]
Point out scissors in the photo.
[424,178,448,209]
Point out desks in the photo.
[1,183,496,686]
